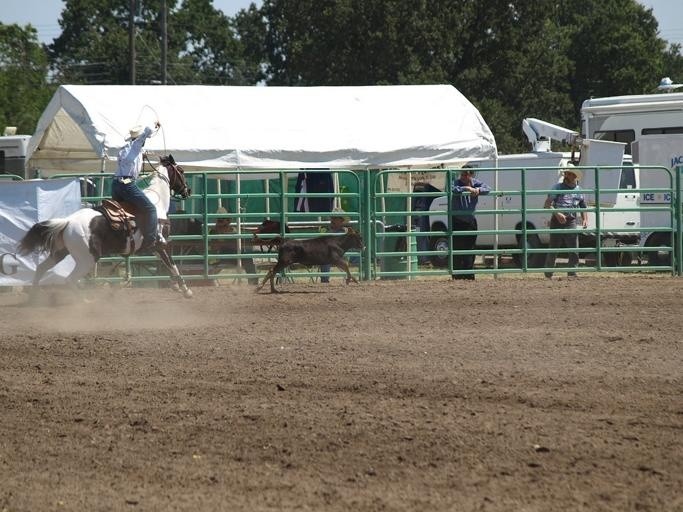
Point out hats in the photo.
[213,207,232,223]
[124,124,145,143]
[330,208,351,224]
[560,165,583,181]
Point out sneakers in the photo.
[145,241,167,253]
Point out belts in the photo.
[113,176,132,181]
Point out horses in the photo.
[17,153,193,298]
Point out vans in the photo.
[426,116,639,268]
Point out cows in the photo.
[253,226,367,293]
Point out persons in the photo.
[413,183,431,266]
[452,165,491,280]
[200,206,259,285]
[321,204,352,283]
[544,164,588,278]
[110,122,161,250]
